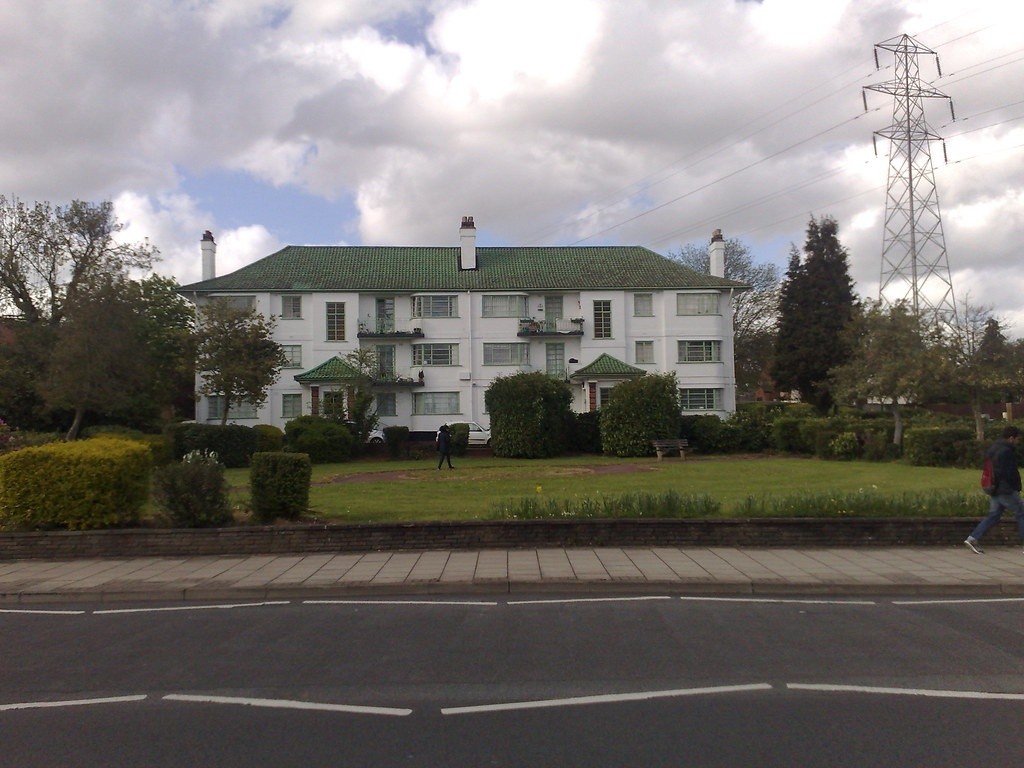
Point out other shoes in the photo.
[449,466,454,469]
[436,466,441,470]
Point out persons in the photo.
[433,424,457,470]
[962,425,1023,557]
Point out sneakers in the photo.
[964,536,987,555]
[1022,547,1024,555]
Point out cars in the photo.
[435,421,491,447]
[342,420,391,444]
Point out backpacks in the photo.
[981,443,1004,498]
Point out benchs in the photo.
[652,439,690,462]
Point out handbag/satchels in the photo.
[435,441,440,451]
